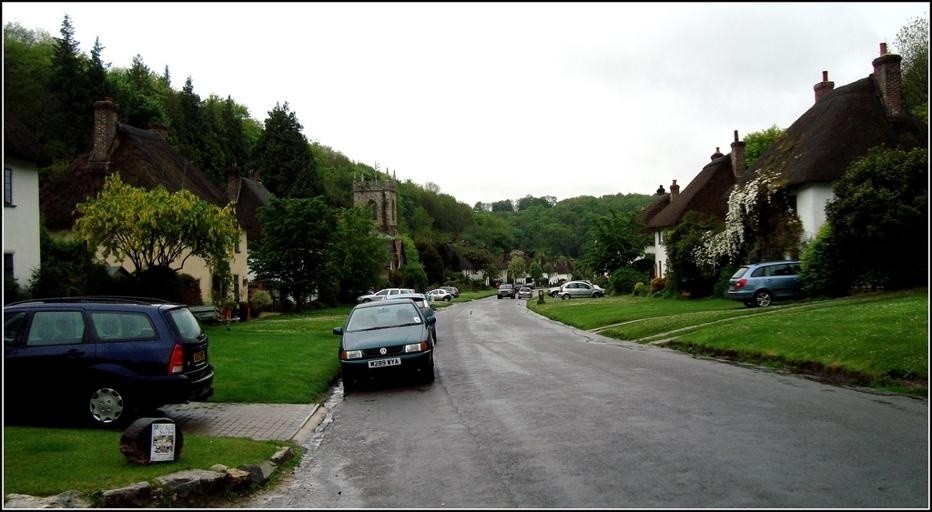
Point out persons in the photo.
[496,280,500,289]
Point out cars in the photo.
[355,283,460,348]
[331,298,439,394]
[496,279,607,301]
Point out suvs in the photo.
[726,260,807,308]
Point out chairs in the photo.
[397,308,411,320]
[102,317,135,339]
[36,318,72,343]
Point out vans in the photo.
[4,291,219,430]
